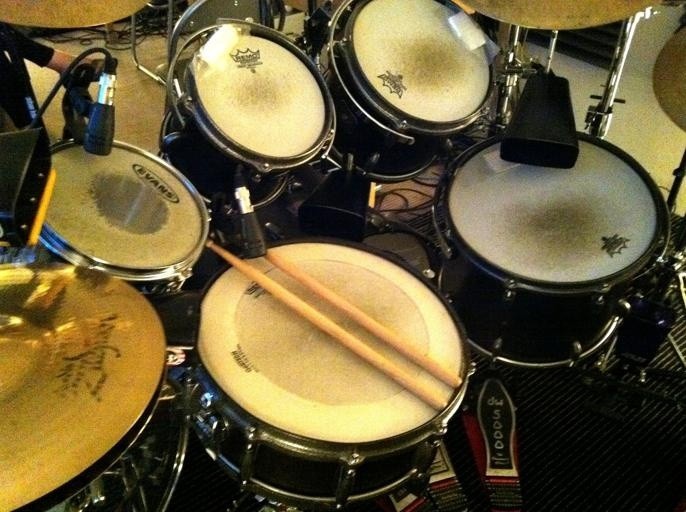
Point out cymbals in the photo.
[0,261,166,512]
[456,0,655,29]
[653,25,686,134]
[0,1,148,29]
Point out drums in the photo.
[311,0,490,184]
[436,130,671,368]
[178,243,467,512]
[166,34,326,211]
[36,138,209,296]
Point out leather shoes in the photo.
[62,57,117,88]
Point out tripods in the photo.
[600,210,686,373]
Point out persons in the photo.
[0,22,119,130]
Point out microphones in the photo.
[234,185,268,258]
[83,58,118,156]
